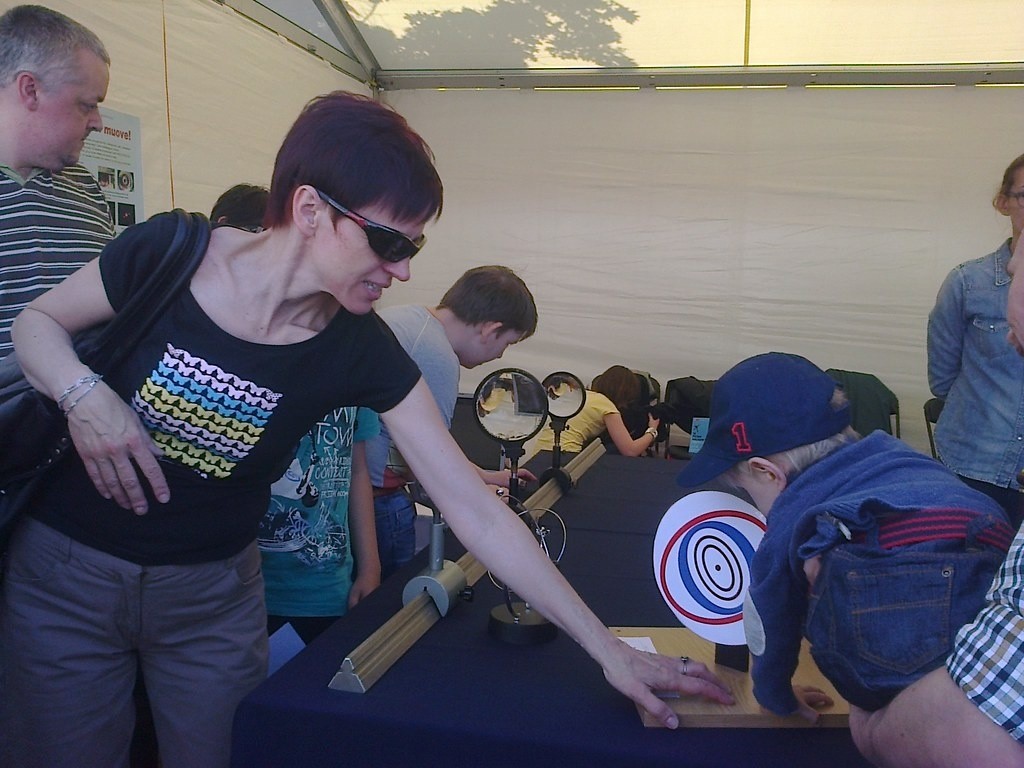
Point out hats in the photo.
[675,351,855,488]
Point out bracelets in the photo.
[645,427,658,440]
[58,373,104,418]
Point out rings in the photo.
[682,656,689,675]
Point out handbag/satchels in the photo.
[1,208,210,553]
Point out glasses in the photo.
[310,188,430,264]
[1004,191,1024,208]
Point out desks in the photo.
[231,451,868,768]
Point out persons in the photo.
[848,227,1024,768]
[209,184,270,234]
[0,5,120,429]
[676,351,1015,722]
[1,90,733,768]
[364,266,539,584]
[505,365,659,472]
[257,406,382,649]
[926,153,1024,533]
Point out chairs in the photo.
[590,369,945,458]
